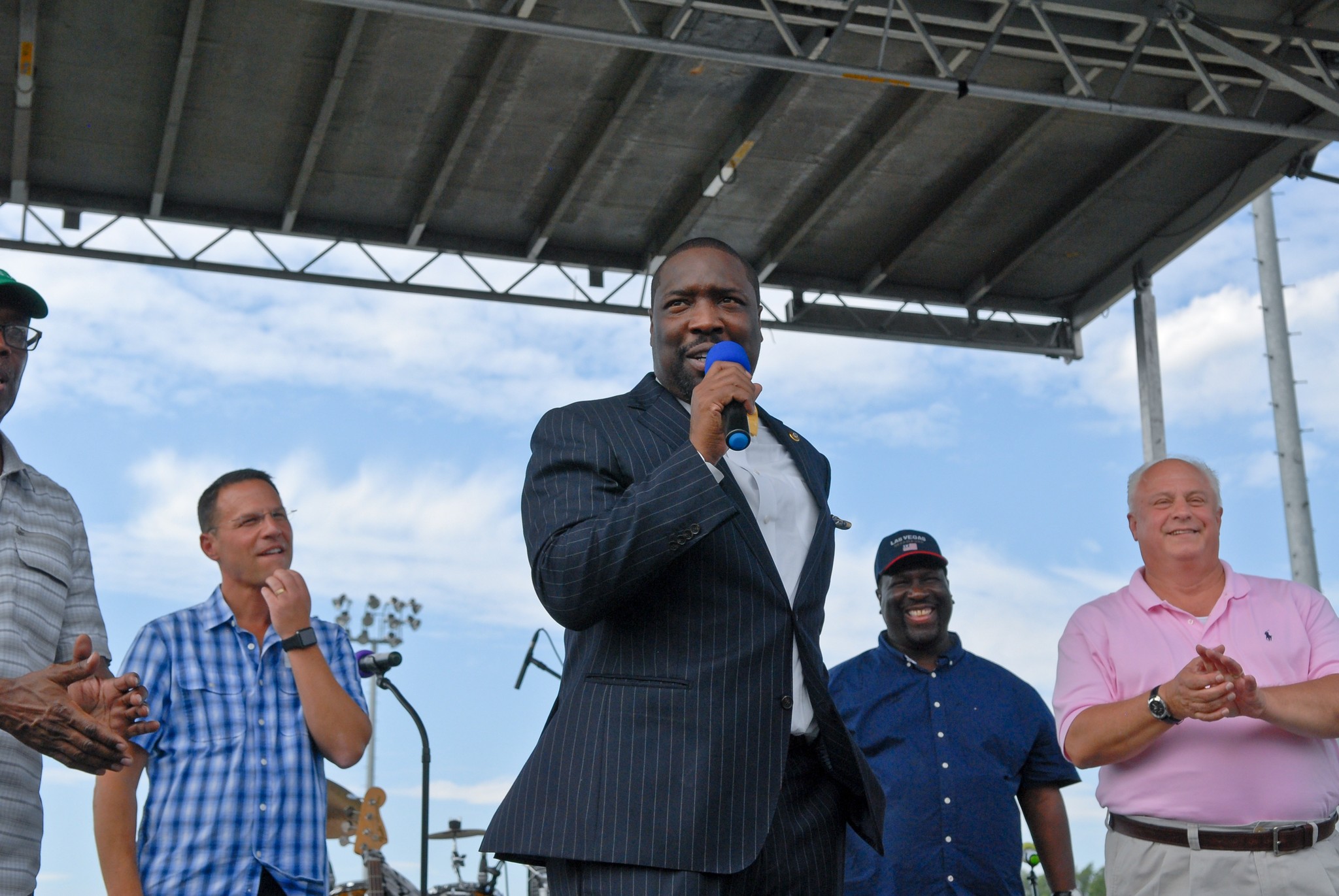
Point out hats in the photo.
[874,530,948,581]
[0,269,48,319]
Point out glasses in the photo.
[0,325,42,351]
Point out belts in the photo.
[1108,812,1338,857]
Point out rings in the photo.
[275,587,286,595]
[1232,671,1243,679]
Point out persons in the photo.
[827,528,1082,896]
[93,467,372,896]
[0,267,161,896]
[478,236,885,896]
[1052,455,1339,896]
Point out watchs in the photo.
[1051,887,1082,896]
[1148,684,1184,725]
[281,627,317,651]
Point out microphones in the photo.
[704,340,752,451]
[516,631,540,689]
[478,852,488,884]
[355,650,402,678]
